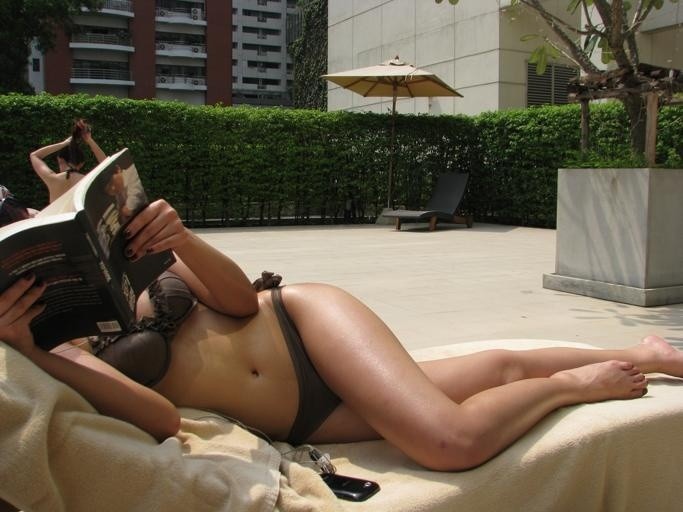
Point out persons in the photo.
[29,119,106,204]
[0,185,683,471]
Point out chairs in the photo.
[382,173,473,232]
[0,338,683,512]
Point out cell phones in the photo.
[315,472,380,502]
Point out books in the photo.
[0,147,178,351]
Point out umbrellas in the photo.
[318,53,464,209]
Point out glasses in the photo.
[0,192,30,227]
